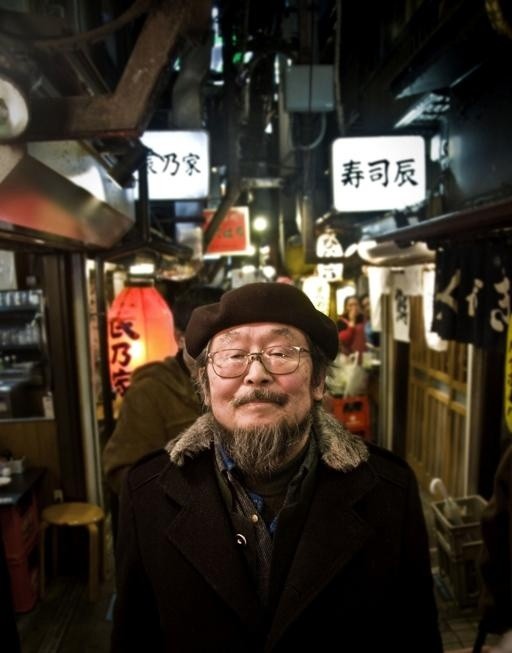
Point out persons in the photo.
[104,283,441,653]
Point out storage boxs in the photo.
[2,488,43,614]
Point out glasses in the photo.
[205,346,309,379]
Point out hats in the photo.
[183,281,339,364]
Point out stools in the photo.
[39,501,106,606]
